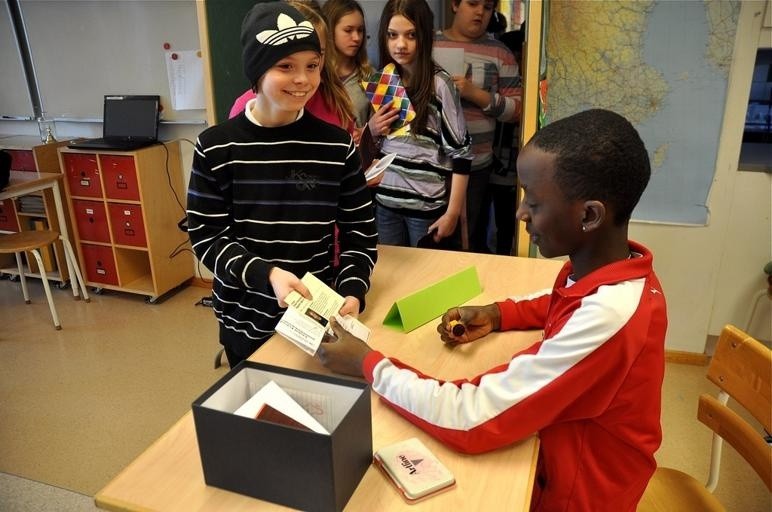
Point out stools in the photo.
[1,229,91,331]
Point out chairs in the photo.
[628,323,771,512]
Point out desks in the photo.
[0,166,81,304]
[93,240,563,512]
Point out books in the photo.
[253,404,314,432]
[236,379,331,436]
[17,195,58,274]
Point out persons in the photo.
[464,9,529,256]
[362,0,476,256]
[306,310,328,327]
[229,1,381,269]
[423,0,524,255]
[182,0,377,371]
[316,0,379,125]
[315,108,668,512]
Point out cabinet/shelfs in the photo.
[0,134,196,305]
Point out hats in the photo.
[242,2,321,86]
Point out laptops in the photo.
[67,94,161,151]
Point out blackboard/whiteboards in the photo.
[196,0,281,128]
[17,0,446,125]
[0,0,37,121]
